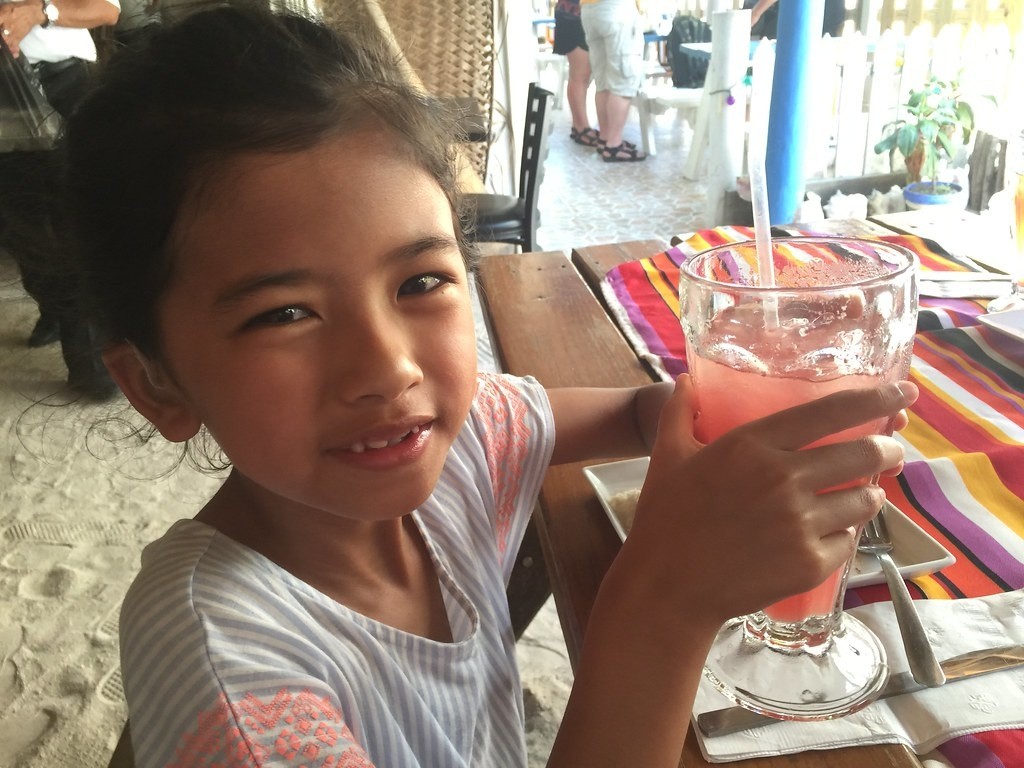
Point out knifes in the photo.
[697,642,1024,738]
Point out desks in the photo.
[678,40,776,75]
[1,97,487,153]
[473,218,1024,768]
[873,204,1024,277]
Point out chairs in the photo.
[454,82,555,254]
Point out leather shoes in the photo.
[29,315,62,347]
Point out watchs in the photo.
[41,0,59,29]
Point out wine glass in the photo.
[679,237,920,721]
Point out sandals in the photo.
[597,138,636,153]
[575,127,599,146]
[602,147,646,162]
[570,127,600,139]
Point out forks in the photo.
[857,506,946,687]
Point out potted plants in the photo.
[873,75,997,210]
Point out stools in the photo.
[532,52,703,155]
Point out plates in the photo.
[581,455,956,599]
[975,309,1024,343]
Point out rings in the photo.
[2,28,9,36]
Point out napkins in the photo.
[691,587,1024,763]
[919,271,1015,298]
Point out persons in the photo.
[742,0,779,42]
[47,6,920,768]
[0,0,167,400]
[581,0,648,162]
[552,0,599,145]
